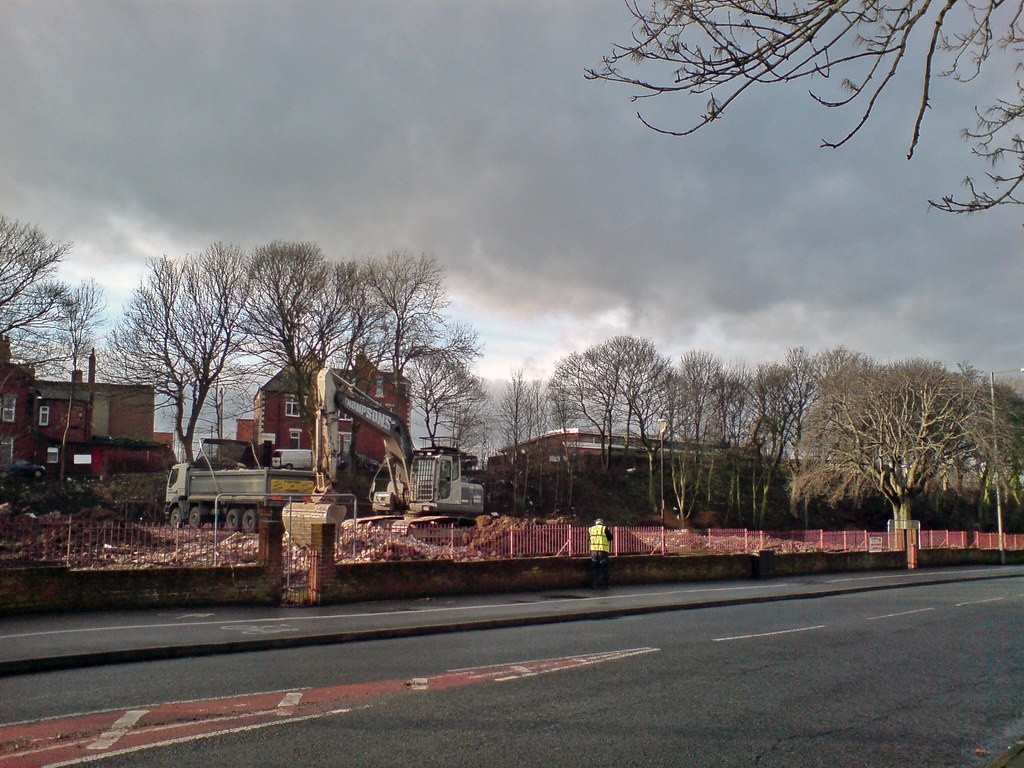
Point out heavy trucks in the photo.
[165,439,319,533]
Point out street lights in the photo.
[659,418,669,554]
[991,367,1024,563]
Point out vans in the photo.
[274,449,314,469]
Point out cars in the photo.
[8,457,47,478]
[340,453,379,471]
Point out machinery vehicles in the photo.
[283,368,486,545]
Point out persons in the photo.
[588,518,613,590]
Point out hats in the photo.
[595,518,603,525]
